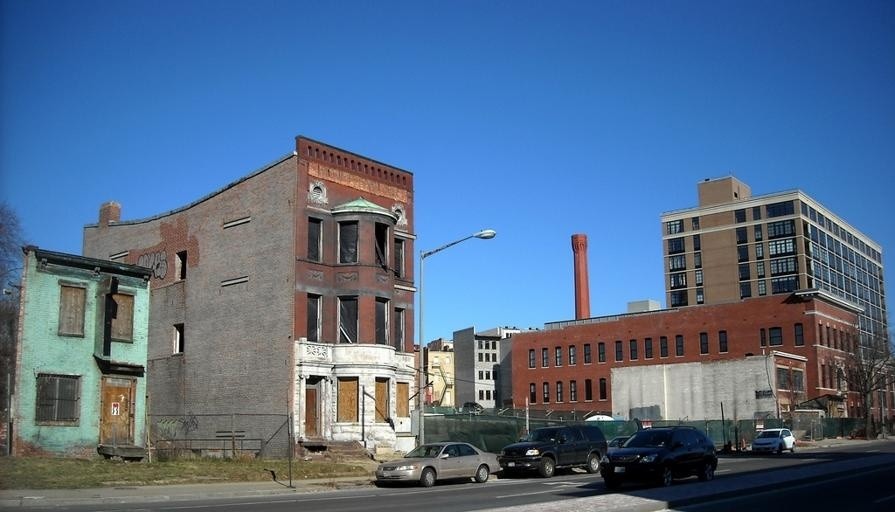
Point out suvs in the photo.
[499,424,607,474]
[600,426,721,480]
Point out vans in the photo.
[461,401,485,415]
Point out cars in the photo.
[375,441,503,489]
[752,427,797,454]
[605,435,630,456]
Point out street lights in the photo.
[417,228,496,455]
